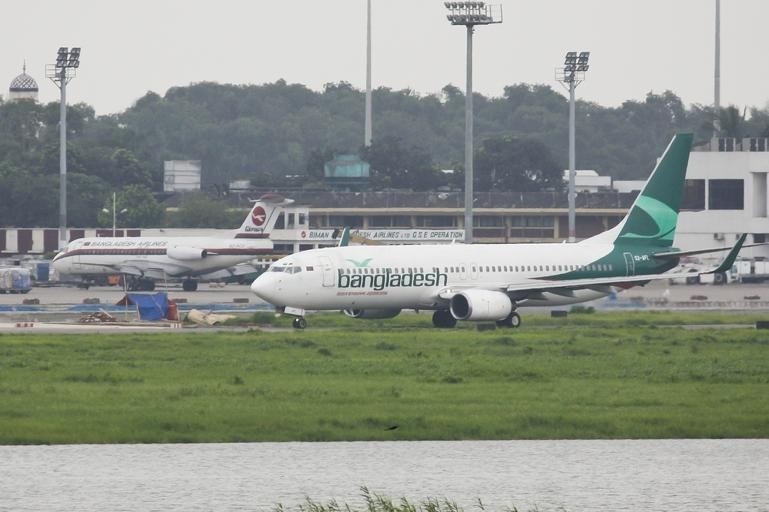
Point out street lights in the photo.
[554,51,593,243]
[44,47,82,251]
[443,0,503,243]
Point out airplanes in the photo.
[47,191,295,291]
[250,132,769,332]
